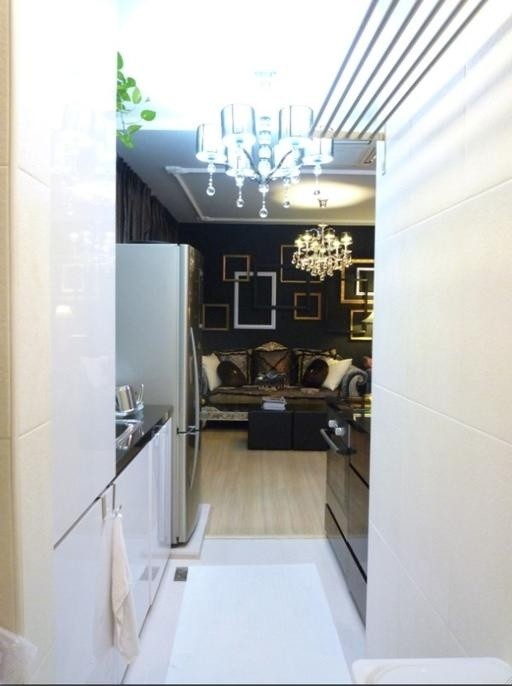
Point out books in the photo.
[258,395,288,411]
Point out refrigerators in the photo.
[115,241,203,548]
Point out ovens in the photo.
[320,400,351,543]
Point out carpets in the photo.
[165,563,350,686]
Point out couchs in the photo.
[200,340,368,432]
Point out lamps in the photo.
[194,66,352,282]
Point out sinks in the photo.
[116,419,142,447]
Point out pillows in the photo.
[201,349,353,391]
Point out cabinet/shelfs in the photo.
[54,444,150,686]
[325,416,372,630]
[148,415,172,604]
[55,48,121,541]
